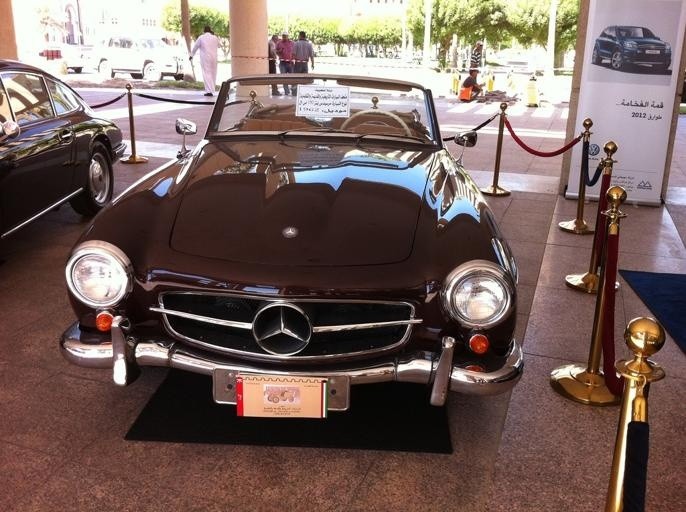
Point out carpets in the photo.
[123,370,455,455]
[618,269,686,354]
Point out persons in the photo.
[277,32,295,95]
[461,70,483,102]
[290,31,314,95]
[269,34,283,96]
[188,25,220,96]
[470,41,483,82]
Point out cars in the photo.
[0,57,127,240]
[57,72,525,422]
[37,41,84,73]
[591,25,672,71]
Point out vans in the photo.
[85,32,187,82]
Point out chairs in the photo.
[241,105,417,139]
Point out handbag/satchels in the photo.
[458,85,474,101]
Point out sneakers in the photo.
[272,90,283,96]
[204,93,214,96]
[285,90,290,95]
